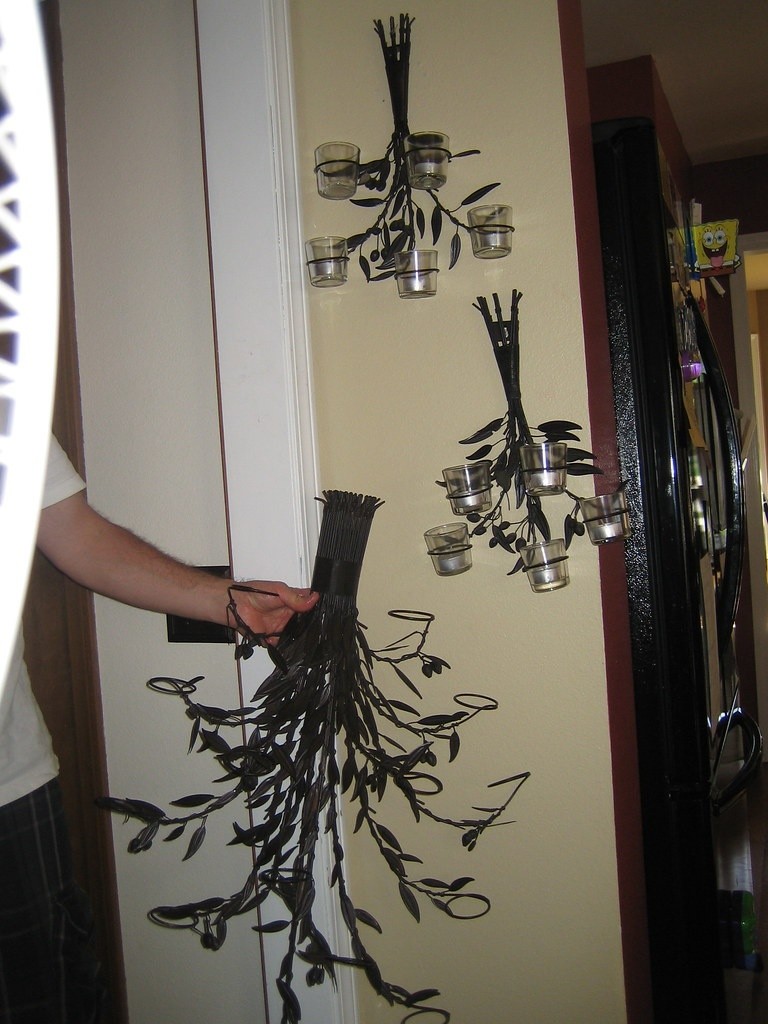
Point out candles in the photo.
[480,231,507,249]
[415,158,439,176]
[314,259,342,278]
[439,543,466,573]
[454,488,484,507]
[529,467,562,487]
[326,175,351,191]
[531,560,561,585]
[402,272,431,295]
[594,514,623,540]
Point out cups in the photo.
[467,204,513,259]
[580,492,632,546]
[442,463,492,516]
[520,538,570,593]
[404,131,449,190]
[520,442,567,496]
[423,522,473,576]
[315,141,361,200]
[305,236,348,288]
[394,250,438,299]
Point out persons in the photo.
[0,431,319,1023]
